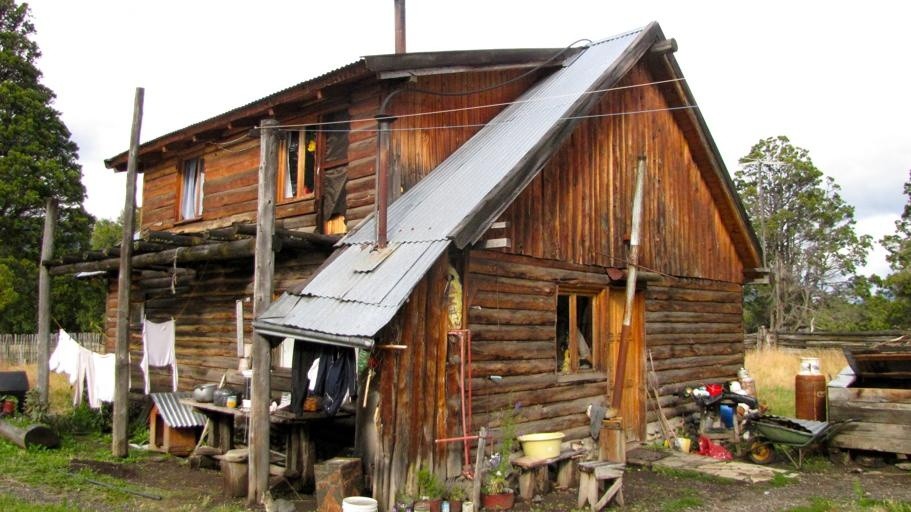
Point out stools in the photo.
[577,459,626,512]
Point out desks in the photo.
[510,446,585,500]
[177,399,353,496]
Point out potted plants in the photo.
[446,487,468,512]
[480,386,517,512]
[415,468,443,512]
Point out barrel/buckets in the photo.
[341,495,378,512]
[796,375,826,421]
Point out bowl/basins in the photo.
[517,433,565,459]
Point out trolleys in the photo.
[741,413,857,472]
